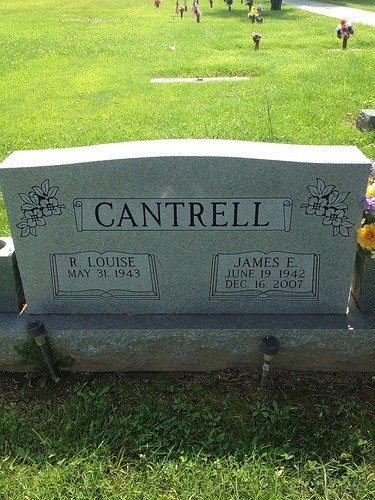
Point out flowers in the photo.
[175,0,254,13]
[180,7,185,18]
[193,3,199,14]
[253,32,262,50]
[196,11,201,23]
[336,19,354,49]
[154,0,162,8]
[248,4,264,24]
